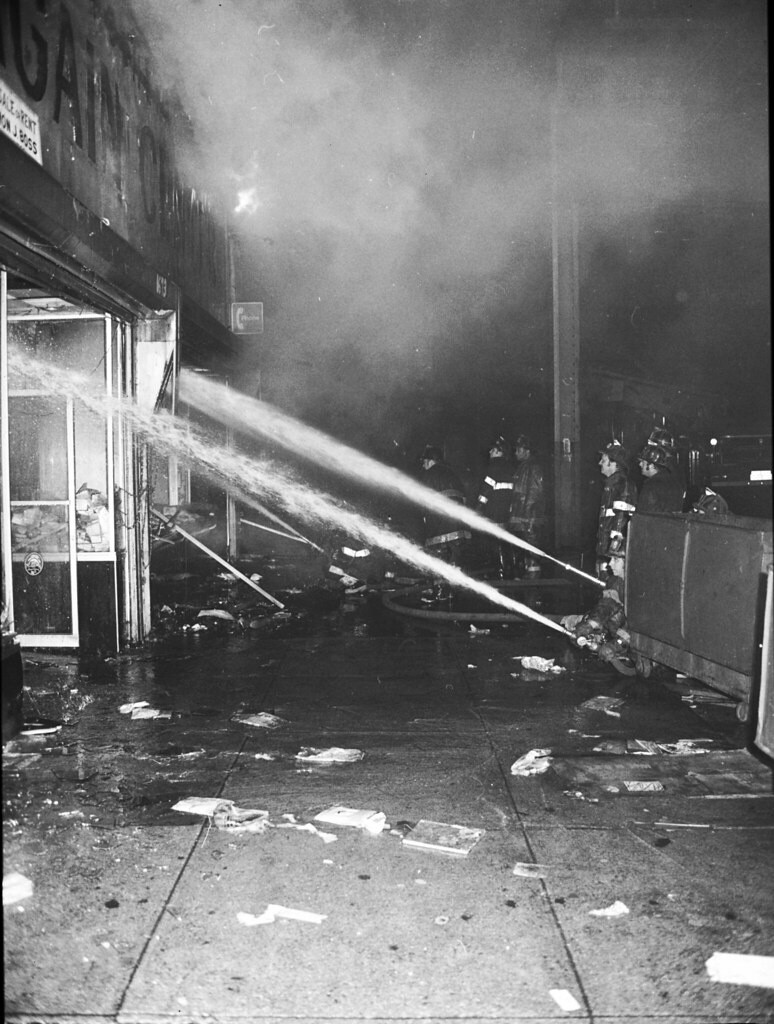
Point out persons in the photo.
[574,430,729,662]
[420,431,546,579]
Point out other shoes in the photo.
[344,581,367,596]
[420,587,453,605]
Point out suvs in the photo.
[610,391,773,504]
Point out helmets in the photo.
[636,446,669,468]
[608,535,624,556]
[599,444,630,468]
[491,435,510,454]
[421,444,442,461]
[648,428,677,453]
[512,434,537,454]
